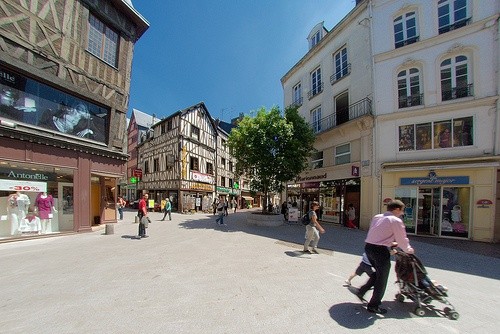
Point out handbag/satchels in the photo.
[218,207,223,211]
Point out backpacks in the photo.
[301,210,315,225]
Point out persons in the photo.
[19,206,41,235]
[345,204,357,231]
[215,199,225,226]
[451,205,465,233]
[35,192,58,234]
[7,191,30,236]
[161,198,172,221]
[268,201,273,213]
[117,195,126,220]
[234,200,238,212]
[224,199,228,216]
[343,249,397,287]
[365,200,414,315]
[303,201,325,254]
[137,193,150,238]
[37,101,105,141]
[281,201,288,221]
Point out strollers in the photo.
[390,244,460,320]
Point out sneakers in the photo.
[345,279,353,287]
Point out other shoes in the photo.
[312,248,319,254]
[215,220,218,224]
[304,249,312,254]
[220,224,224,226]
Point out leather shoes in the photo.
[367,303,388,314]
[356,292,364,301]
